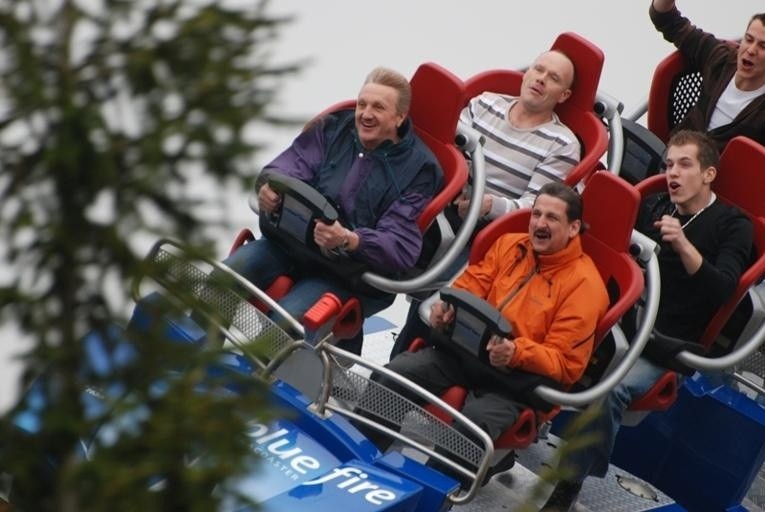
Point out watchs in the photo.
[339,228,350,249]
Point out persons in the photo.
[480,128,755,512]
[334,50,583,367]
[357,181,611,480]
[189,67,446,368]
[648,0,765,157]
